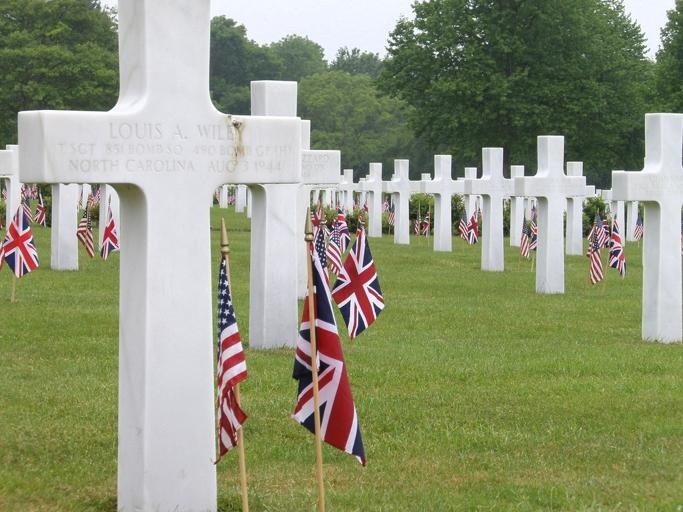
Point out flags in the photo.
[0,238,4,272]
[414,210,421,235]
[310,199,394,285]
[331,226,385,339]
[467,209,479,246]
[458,210,468,240]
[289,242,365,466]
[585,207,643,286]
[212,254,249,464]
[0,183,119,261]
[215,189,234,206]
[4,205,40,279]
[519,204,537,260]
[422,208,429,238]
